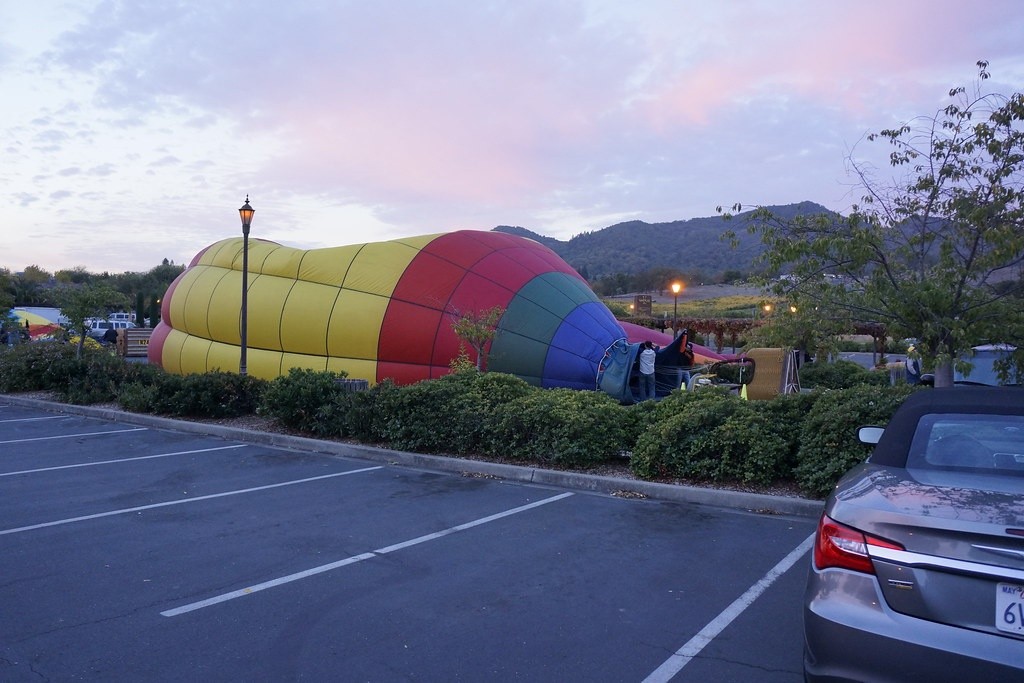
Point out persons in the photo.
[906,347,921,385]
[638,341,657,398]
[677,342,694,391]
[102,326,119,352]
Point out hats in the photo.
[687,341,693,348]
[908,347,915,353]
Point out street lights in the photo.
[671,280,680,339]
[238,192,256,389]
[764,305,773,327]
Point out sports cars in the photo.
[797,381,1024,683]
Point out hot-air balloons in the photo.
[0,308,117,359]
[147,229,757,416]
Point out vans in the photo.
[86,320,139,340]
[107,313,136,323]
